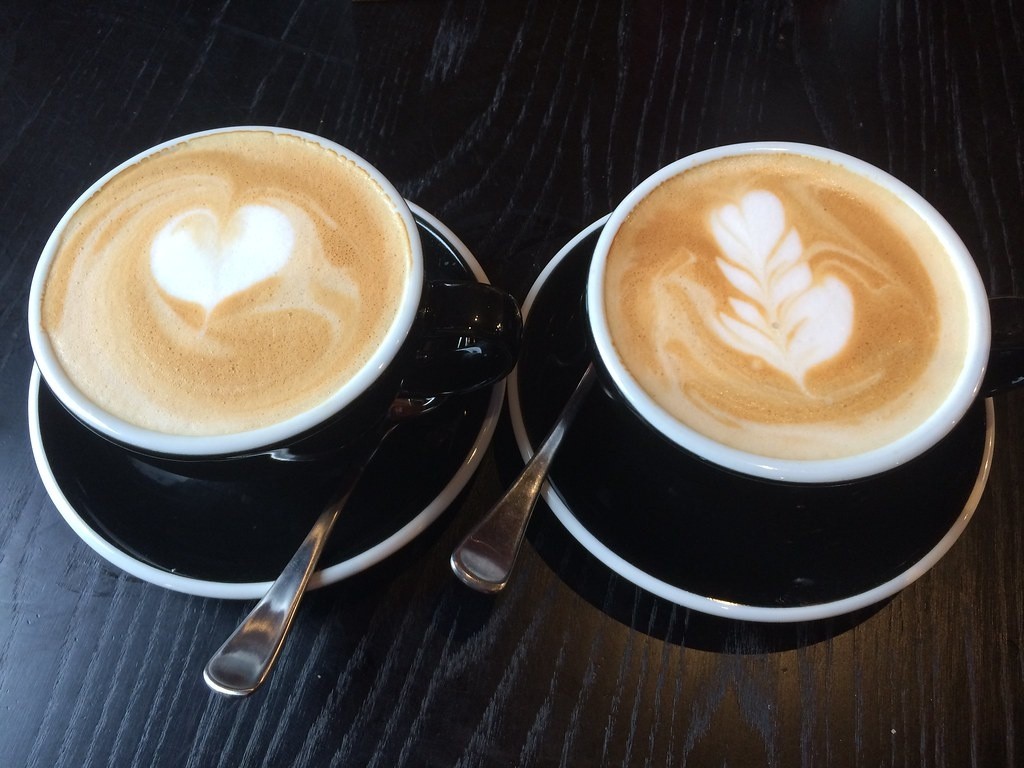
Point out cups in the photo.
[28,126,523,460]
[587,141,1024,484]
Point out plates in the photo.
[507,212,995,622]
[28,198,506,599]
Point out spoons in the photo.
[202,395,458,696]
